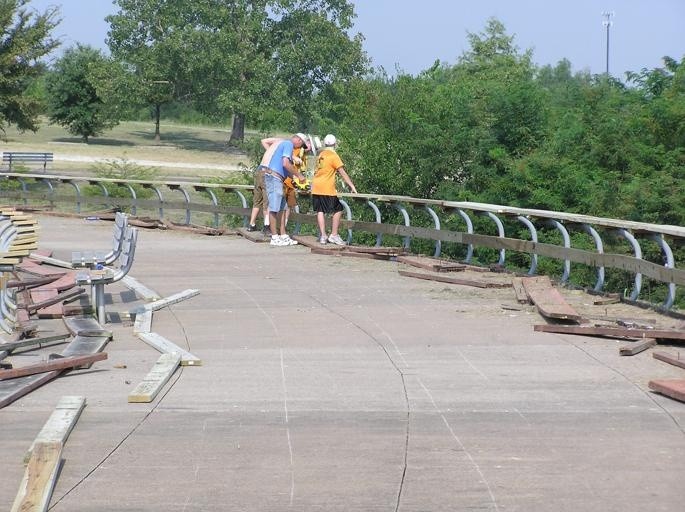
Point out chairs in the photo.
[2,151,53,171]
[71,211,138,327]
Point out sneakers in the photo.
[248,224,259,232]
[263,225,298,245]
[320,234,346,245]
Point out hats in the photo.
[295,132,336,157]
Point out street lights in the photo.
[601,10,615,72]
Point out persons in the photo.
[310,134,358,246]
[246,138,284,237]
[265,132,307,246]
[285,132,322,236]
